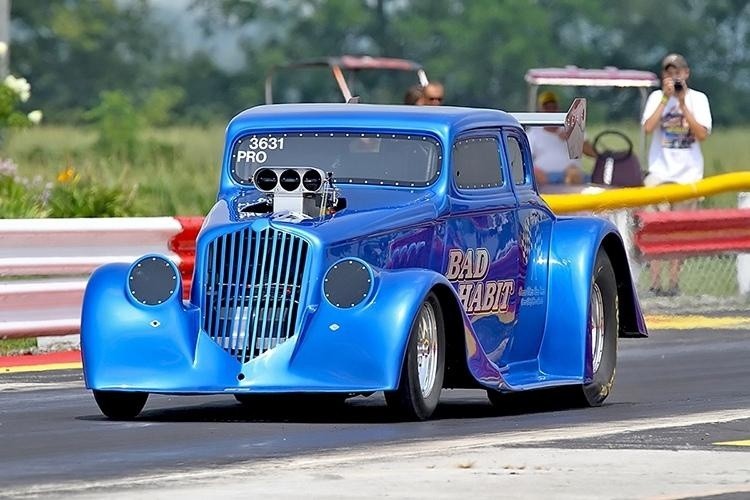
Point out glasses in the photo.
[428,97,441,100]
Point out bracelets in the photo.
[661,93,671,106]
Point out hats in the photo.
[538,91,558,105]
[662,55,688,68]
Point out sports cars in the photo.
[81,97,648,423]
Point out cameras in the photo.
[674,78,683,93]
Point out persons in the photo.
[423,81,445,106]
[405,85,424,106]
[641,53,713,296]
[524,91,598,185]
